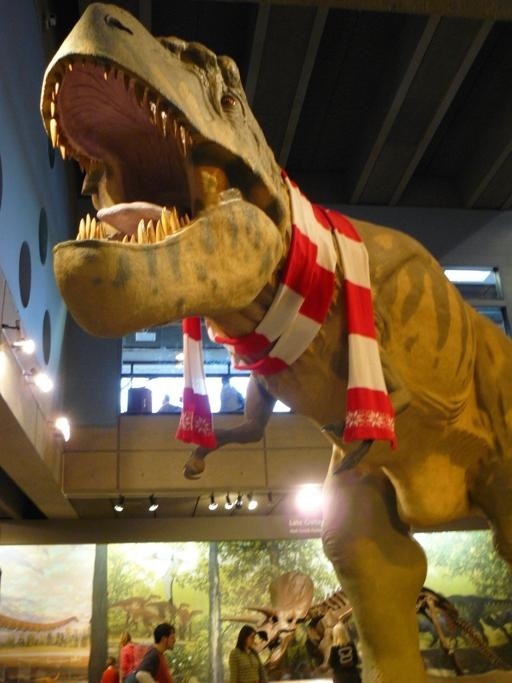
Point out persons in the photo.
[101,657,119,683]
[229,624,267,682]
[118,631,138,682]
[319,622,362,683]
[156,394,181,413]
[255,630,272,682]
[218,376,245,412]
[135,623,176,682]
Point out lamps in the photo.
[51,411,73,446]
[146,492,157,514]
[13,337,34,355]
[206,492,260,512]
[136,331,156,343]
[113,494,125,513]
[174,334,183,367]
[31,371,56,393]
